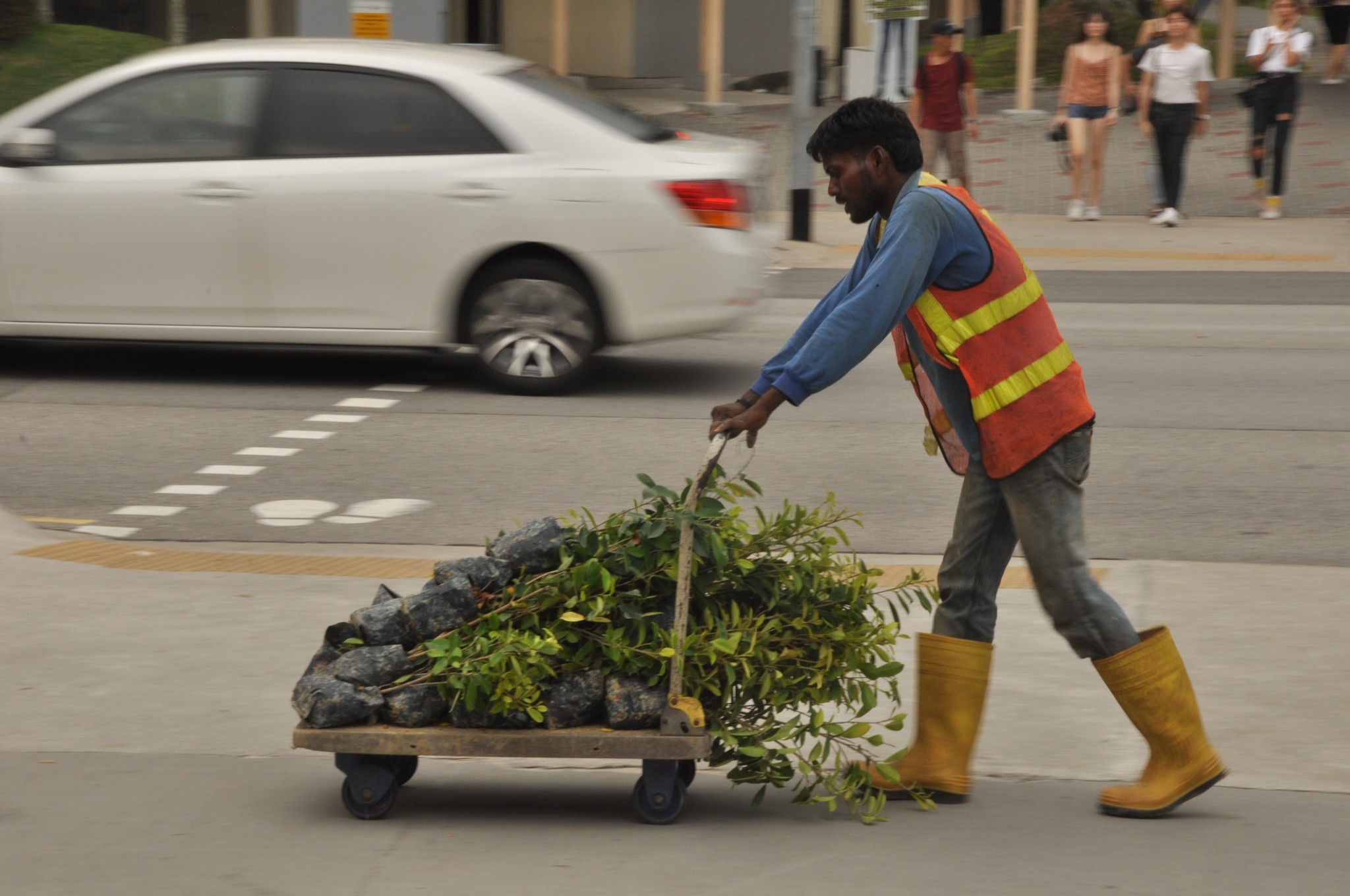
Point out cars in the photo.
[0,37,776,401]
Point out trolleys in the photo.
[292,429,711,825]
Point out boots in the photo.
[1092,624,1232,817]
[847,631,994,805]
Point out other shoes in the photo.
[1085,207,1102,220]
[1259,196,1283,220]
[1249,178,1267,201]
[1067,200,1085,220]
[1149,207,1179,226]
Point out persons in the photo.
[1245,0,1313,219]
[1120,0,1201,116]
[1314,0,1350,85]
[709,96,1229,814]
[1137,5,1216,227]
[912,19,980,193]
[1054,6,1122,221]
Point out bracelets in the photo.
[1107,106,1118,110]
[1196,115,1209,121]
[735,399,752,409]
[966,119,977,123]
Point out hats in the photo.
[930,19,966,36]
